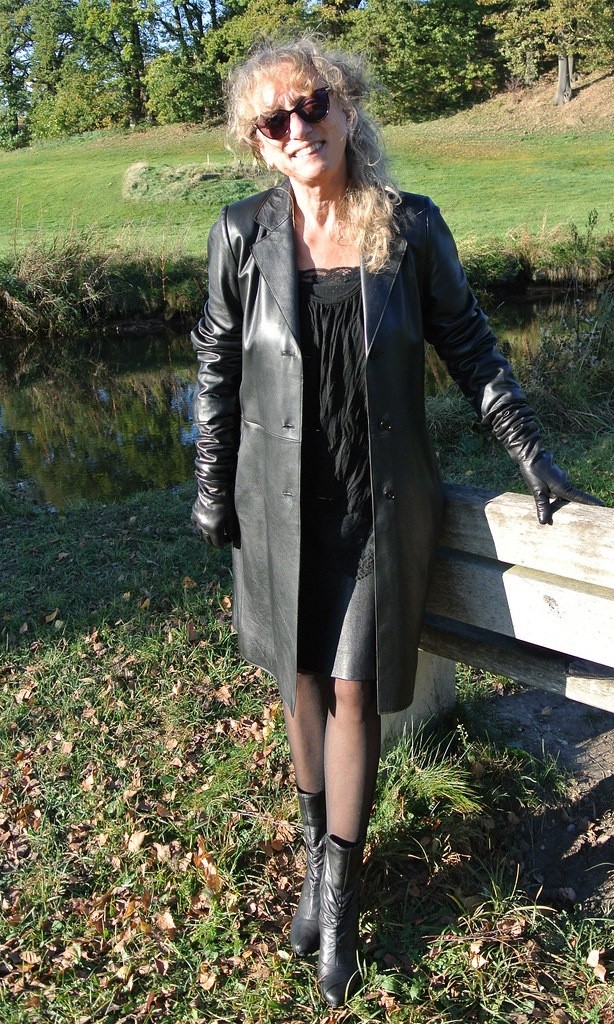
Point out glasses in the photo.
[252,86,331,140]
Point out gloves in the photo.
[491,403,605,524]
[192,440,242,549]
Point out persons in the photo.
[189,45,607,1006]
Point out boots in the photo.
[290,783,327,957]
[317,833,367,1007]
